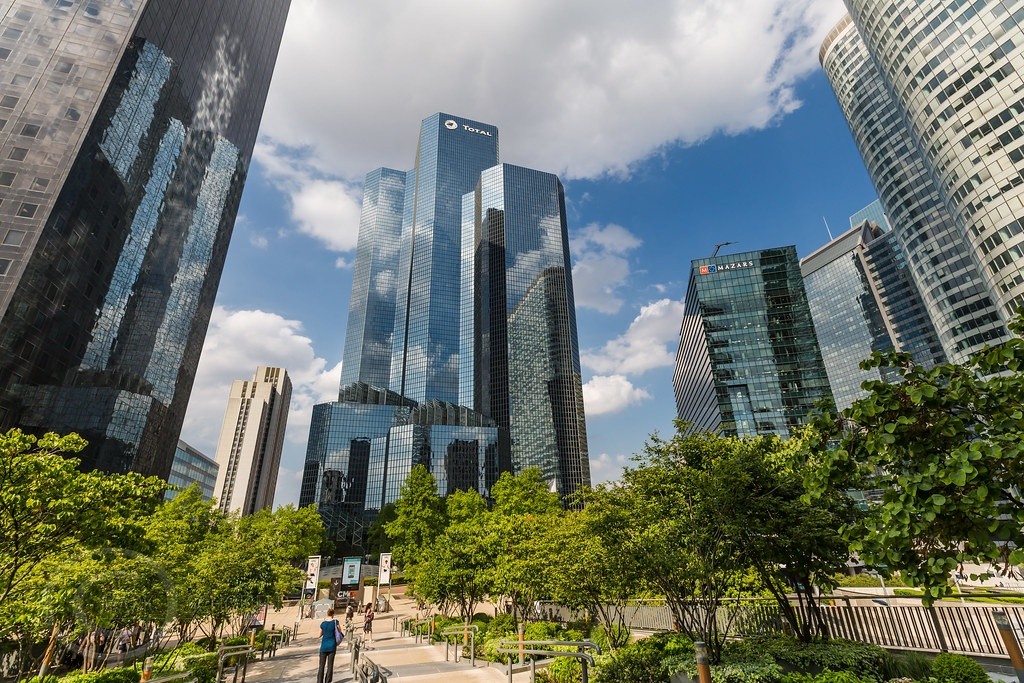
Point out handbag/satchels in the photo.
[363,618,372,632]
[334,619,344,645]
[349,608,353,618]
[363,613,374,621]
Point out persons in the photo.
[317,609,342,683]
[356,603,361,615]
[77,626,132,670]
[363,602,376,652]
[995,582,1004,587]
[345,603,353,621]
[382,560,390,572]
[309,563,318,576]
